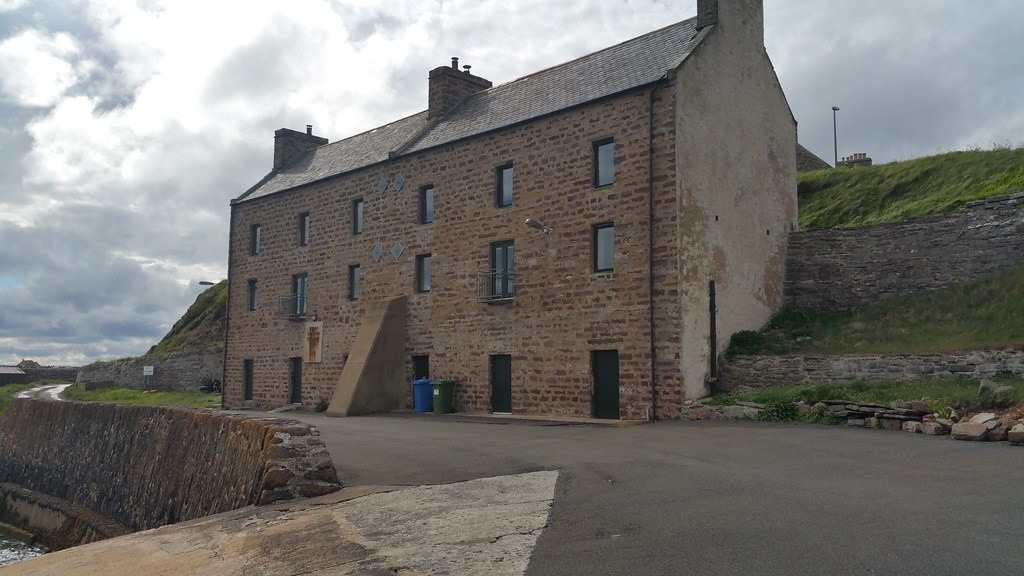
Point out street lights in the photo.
[832,105,840,168]
[198,280,232,409]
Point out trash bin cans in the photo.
[429,379,457,414]
[412,379,433,412]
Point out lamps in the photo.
[524,218,553,234]
[199,281,229,287]
